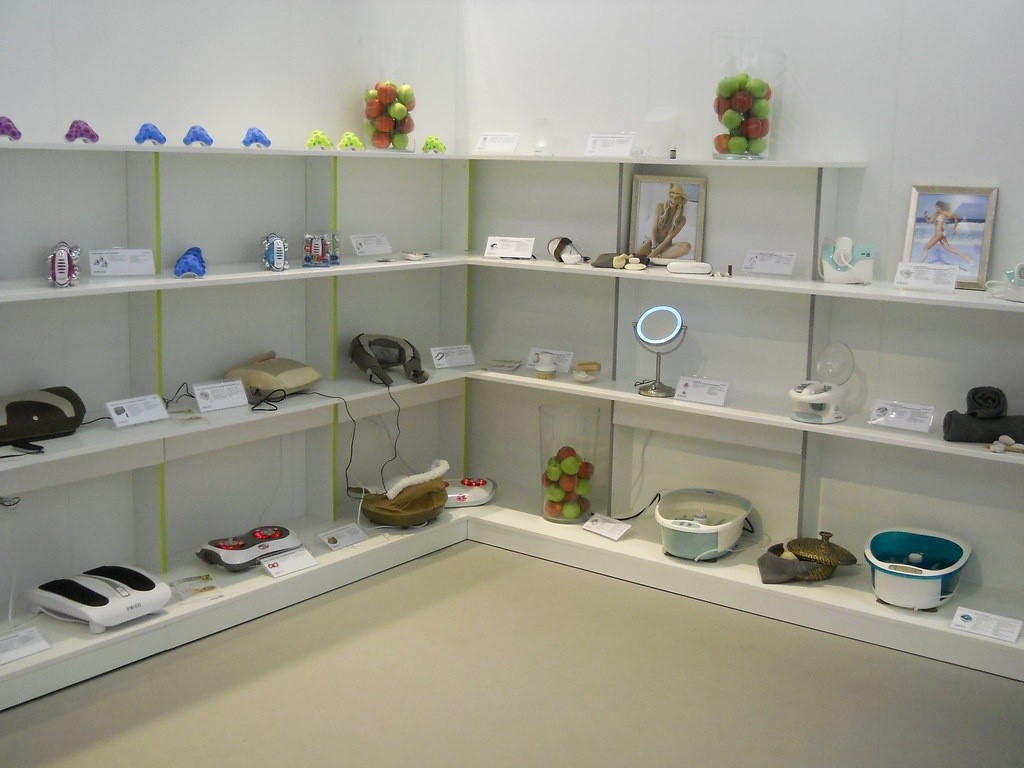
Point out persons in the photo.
[918,201,976,268]
[632,183,691,259]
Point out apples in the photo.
[365,80,414,149]
[714,73,770,155]
[542,446,593,519]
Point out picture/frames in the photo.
[903,184,999,291]
[628,175,707,266]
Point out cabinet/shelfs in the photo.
[0,138,1024,713]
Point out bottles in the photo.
[534,352,557,380]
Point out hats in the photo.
[668,184,686,196]
